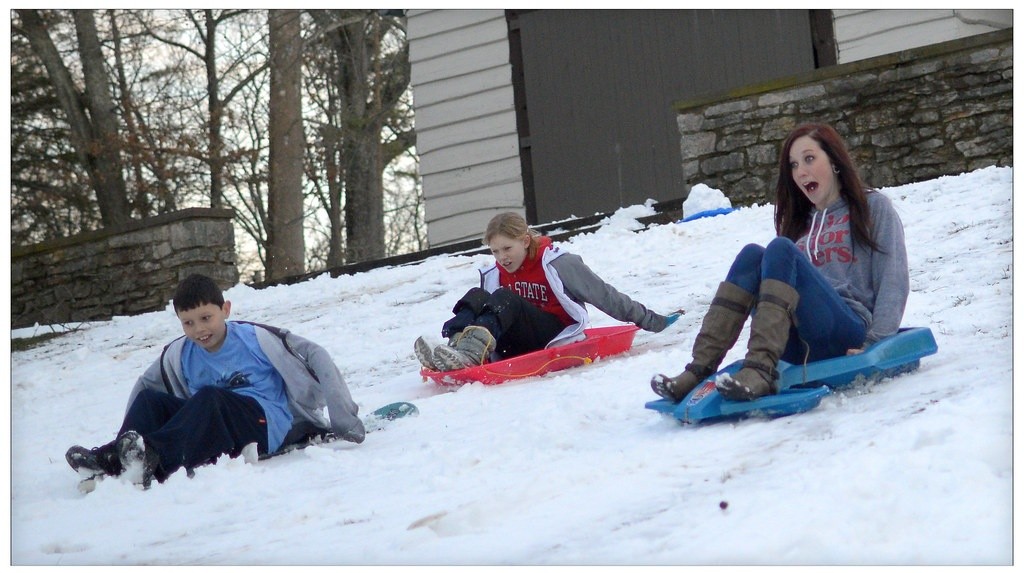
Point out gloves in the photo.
[666,310,684,328]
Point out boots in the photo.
[431,326,496,371]
[414,332,463,370]
[716,279,800,401]
[651,280,755,405]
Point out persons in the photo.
[653,123,909,399]
[66,274,364,490]
[413,213,688,371]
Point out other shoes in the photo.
[65,442,121,481]
[116,431,153,490]
